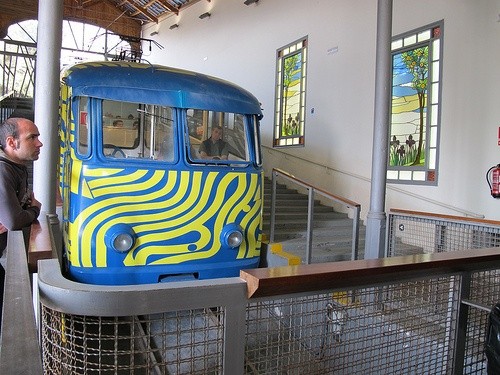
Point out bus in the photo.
[56,32,264,285]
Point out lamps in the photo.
[169,23,178,29]
[199,12,210,19]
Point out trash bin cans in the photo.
[485,303,500,375]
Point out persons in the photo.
[0,117,43,346]
[102,109,173,149]
[198,124,230,162]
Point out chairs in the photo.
[190,144,200,160]
